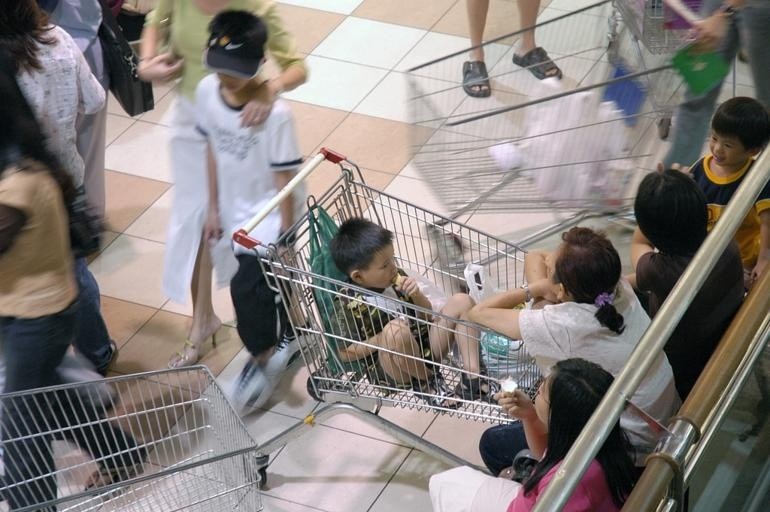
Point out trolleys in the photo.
[232,148,546,489]
[405,0,729,294]
[0,365,264,512]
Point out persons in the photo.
[428,96,769,511]
[135,0,309,372]
[661,1,770,172]
[333,218,510,412]
[0,1,150,512]
[462,2,563,97]
[189,9,324,420]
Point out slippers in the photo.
[513,47,564,80]
[462,62,490,99]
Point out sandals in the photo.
[457,375,503,404]
[411,370,464,411]
[85,465,137,490]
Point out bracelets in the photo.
[278,232,296,246]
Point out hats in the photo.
[204,9,266,79]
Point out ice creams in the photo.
[501,379,518,398]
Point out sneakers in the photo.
[238,323,316,410]
[95,339,117,372]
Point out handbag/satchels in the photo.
[97,18,155,116]
[67,210,101,256]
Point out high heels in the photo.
[168,327,222,369]
